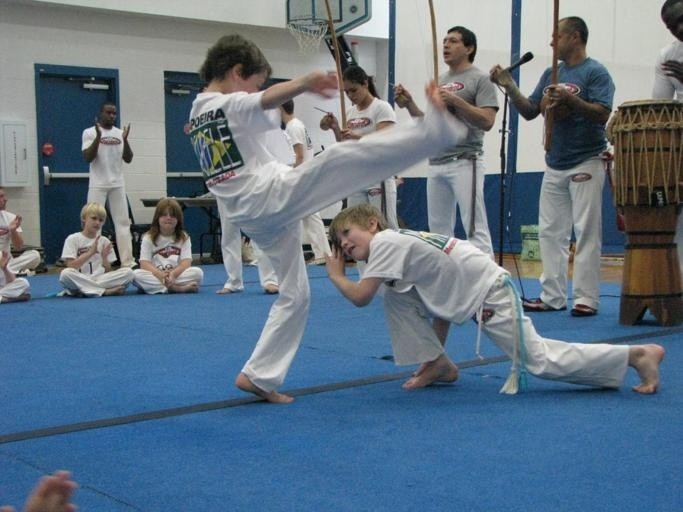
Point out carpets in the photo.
[0,259,683,512]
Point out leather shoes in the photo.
[573,303,597,315]
[521,298,568,310]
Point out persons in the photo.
[491,16,614,315]
[390,25,499,259]
[323,205,664,394]
[1,472,80,512]
[215,194,279,292]
[279,100,331,265]
[260,127,295,167]
[188,34,466,405]
[1,188,38,274]
[653,1,683,101]
[133,199,203,293]
[320,66,397,281]
[0,250,31,303]
[59,204,133,296]
[82,102,137,270]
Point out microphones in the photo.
[491,52,534,80]
